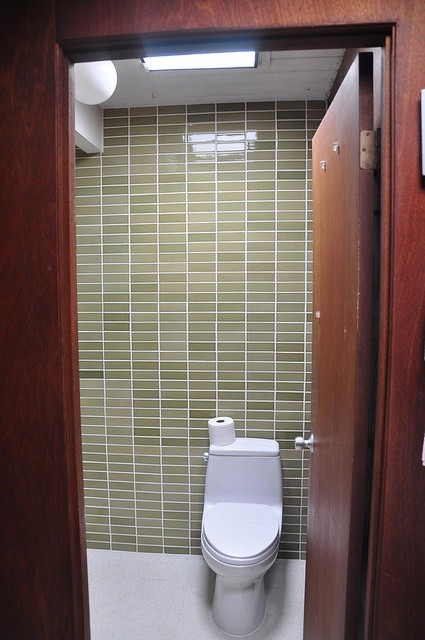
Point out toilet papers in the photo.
[208,416,236,446]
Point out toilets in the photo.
[200,437,285,638]
